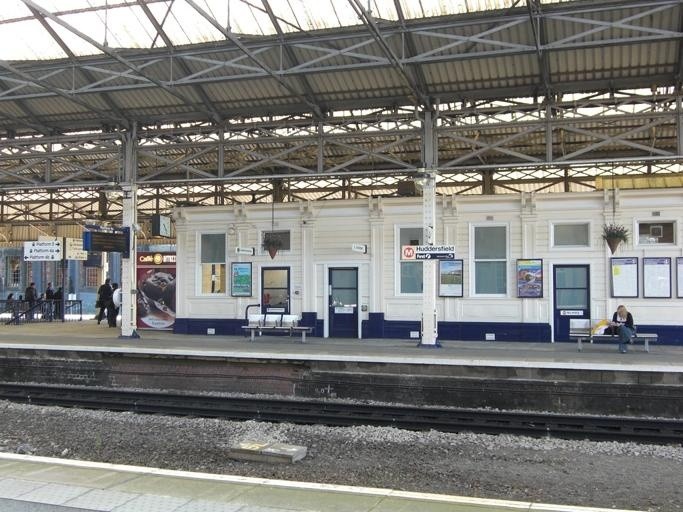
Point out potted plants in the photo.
[602,223,631,254]
[262,232,284,258]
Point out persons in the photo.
[109,283,121,329]
[4,280,63,325]
[96,276,112,325]
[610,304,634,354]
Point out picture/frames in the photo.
[609,257,683,299]
[515,259,543,297]
[439,259,463,297]
[231,262,252,297]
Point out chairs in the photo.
[241,314,317,344]
[569,319,657,353]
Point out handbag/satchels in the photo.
[592,320,616,335]
[95,301,101,308]
